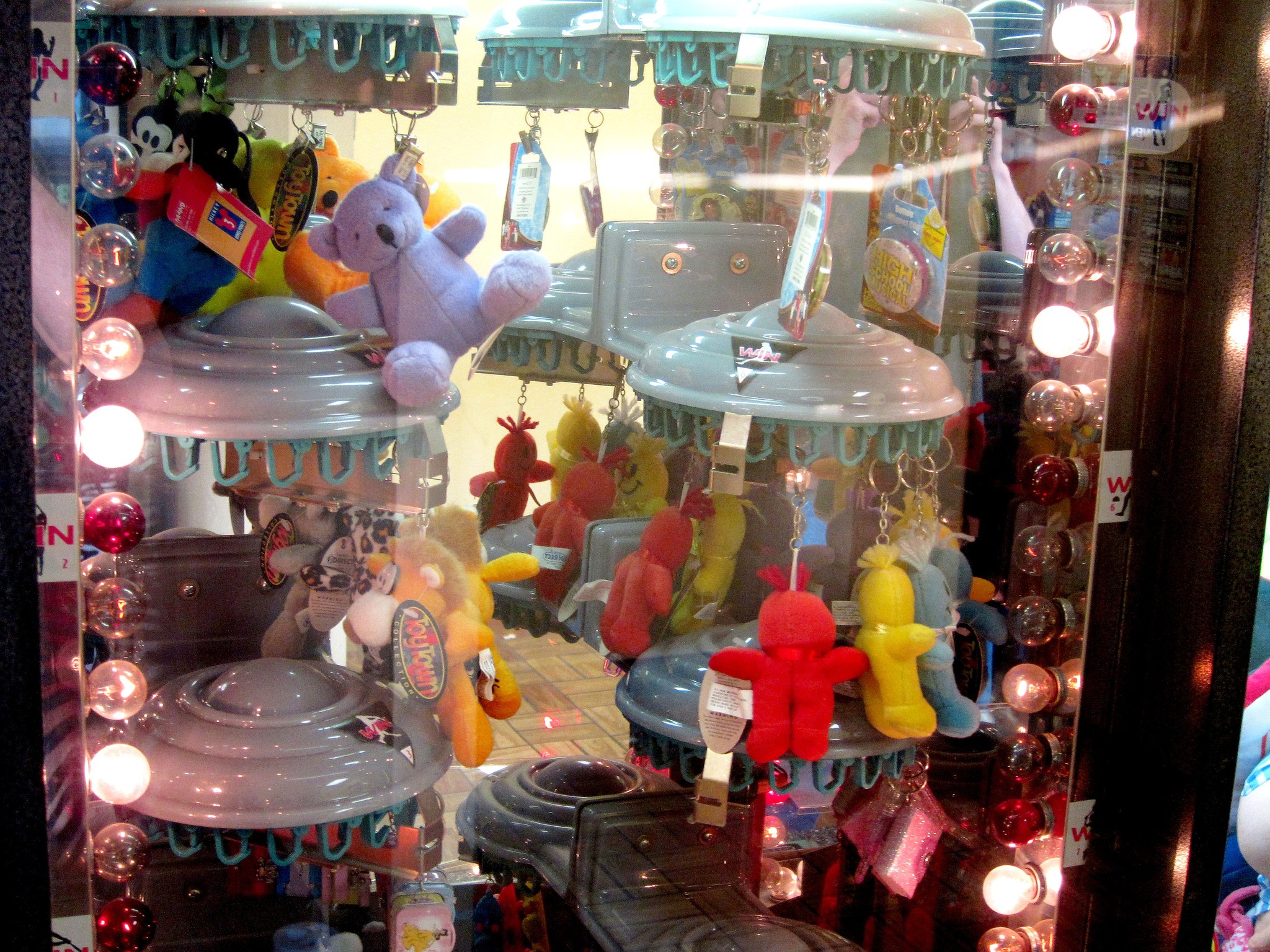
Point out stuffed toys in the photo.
[77,99,259,335]
[213,476,540,769]
[470,398,805,658]
[308,152,549,405]
[712,487,978,752]
[284,136,464,314]
[198,134,294,314]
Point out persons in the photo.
[821,41,1034,425]
